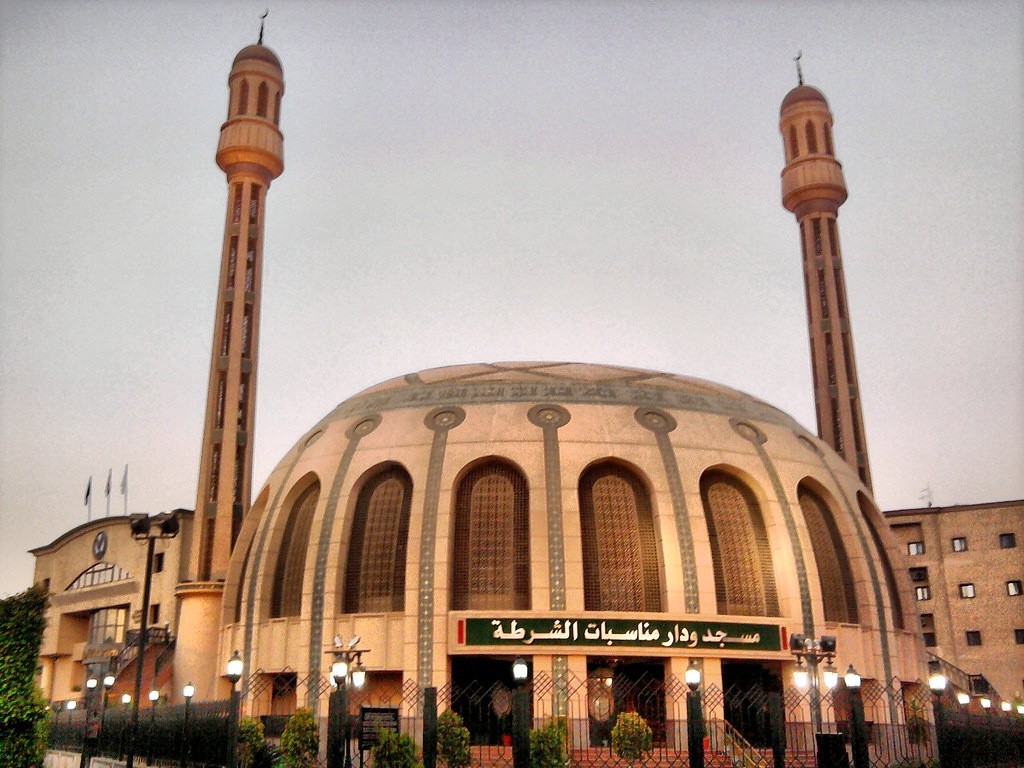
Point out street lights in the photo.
[845,664,869,768]
[182,681,194,768]
[97,674,115,757]
[227,650,244,768]
[686,663,703,768]
[928,671,1024,768]
[510,658,532,766]
[791,634,839,734]
[65,700,77,752]
[79,676,97,768]
[146,688,160,766]
[119,694,132,761]
[323,648,371,768]
